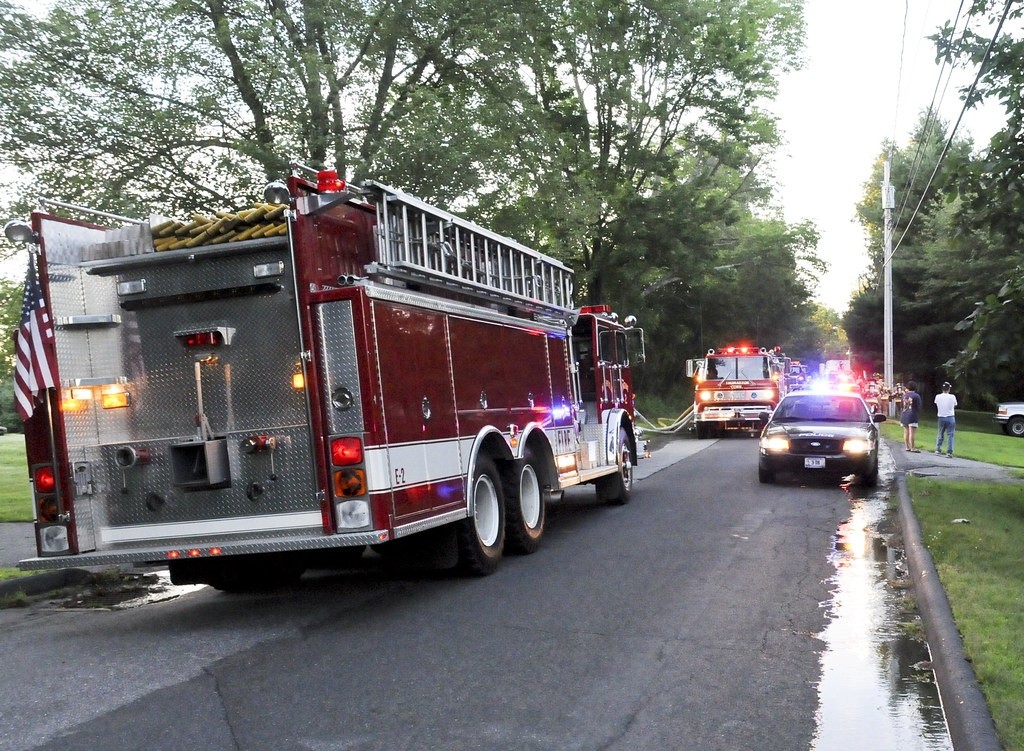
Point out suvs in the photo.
[992,401,1024,438]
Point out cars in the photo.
[757,392,887,491]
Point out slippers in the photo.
[906,449,911,452]
[911,449,921,453]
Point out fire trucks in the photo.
[1,160,645,595]
[684,345,880,439]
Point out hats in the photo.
[942,381,951,387]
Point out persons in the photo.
[898,381,922,453]
[934,381,958,458]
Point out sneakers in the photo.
[945,453,952,458]
[934,450,941,455]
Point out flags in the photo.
[11,252,58,421]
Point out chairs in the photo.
[786,401,857,418]
